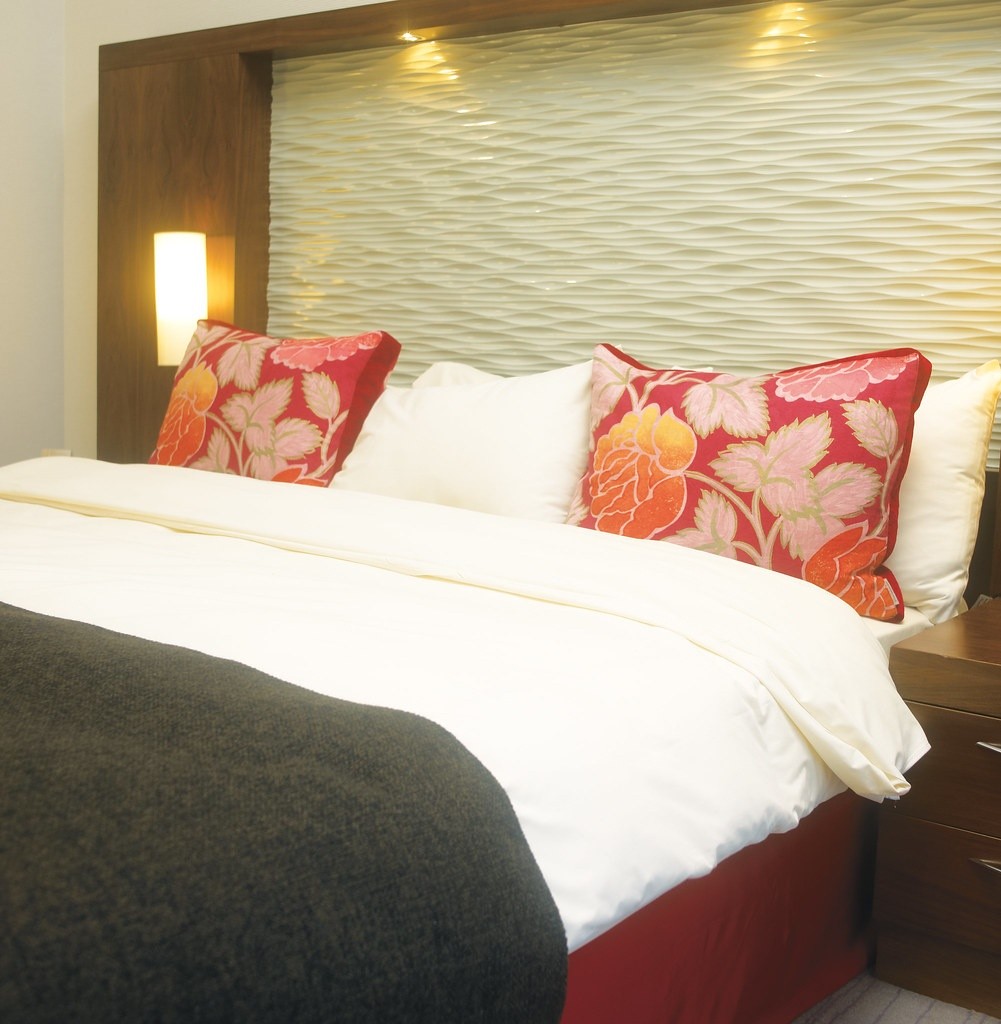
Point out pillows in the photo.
[329,343,623,525]
[564,343,933,625]
[882,359,1001,624]
[148,319,401,487]
[412,360,712,389]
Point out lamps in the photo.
[153,232,209,367]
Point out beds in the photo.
[0,456,969,1024]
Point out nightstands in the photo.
[873,596,1001,1020]
